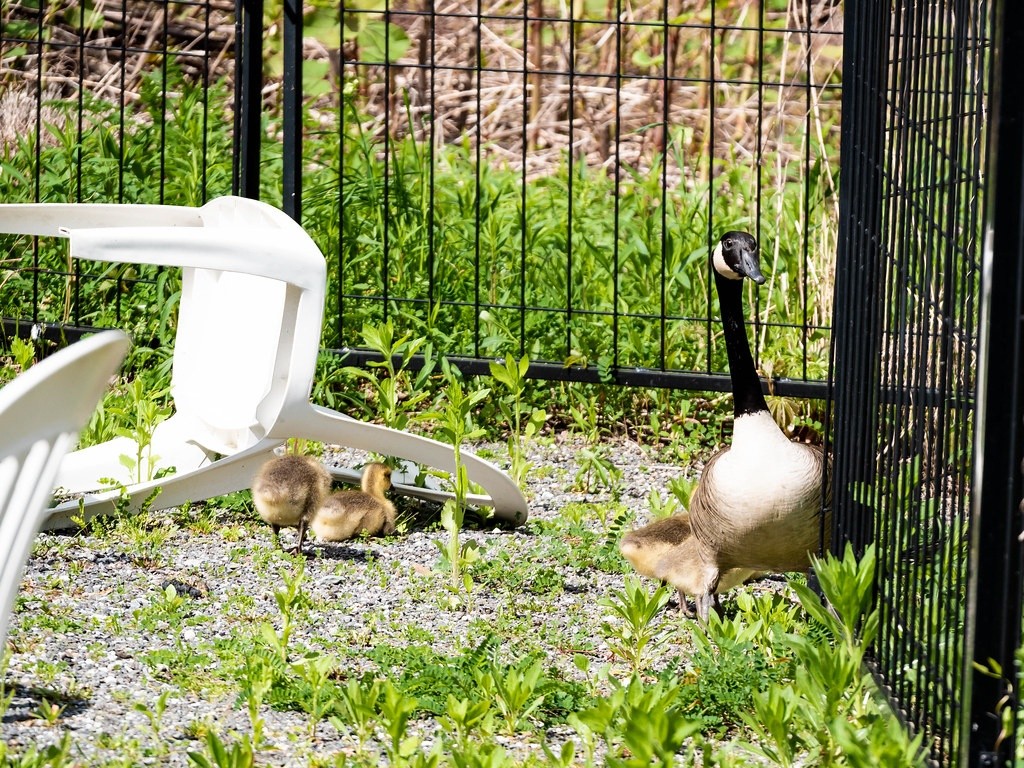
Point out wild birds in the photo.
[310,463,397,542]
[252,456,332,558]
[686,231,834,604]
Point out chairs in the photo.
[0,196,528,534]
[0,330,129,644]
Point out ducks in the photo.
[614,516,756,612]
